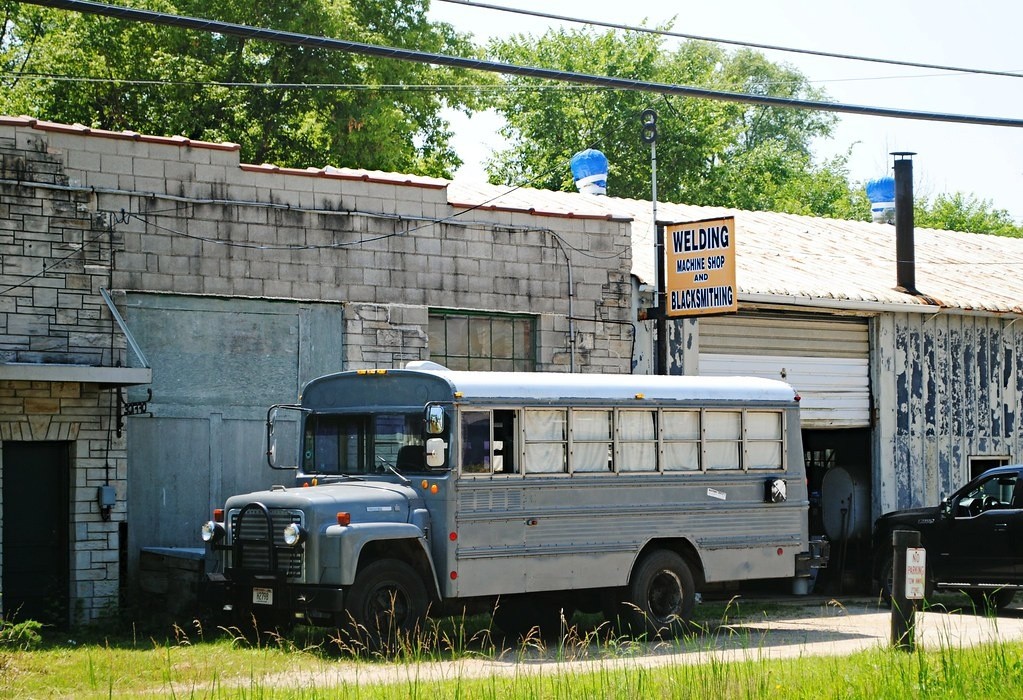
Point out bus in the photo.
[197,364,858,606]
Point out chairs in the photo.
[395,444,429,472]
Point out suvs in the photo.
[870,466,1023,605]
